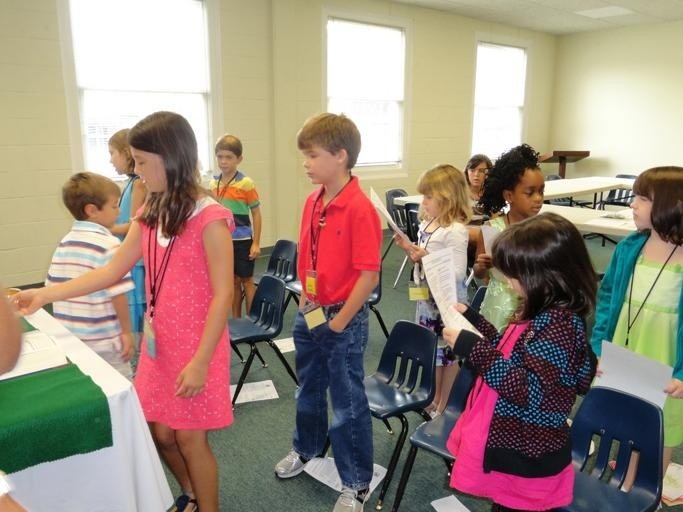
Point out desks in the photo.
[504,201,638,236]
[0,288,178,512]
[393,177,635,247]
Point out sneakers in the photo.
[274,452,326,479]
[332,486,368,512]
[423,403,441,420]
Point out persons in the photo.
[474,144,544,336]
[583,166,683,512]
[462,154,493,267]
[274,112,382,512]
[392,164,474,419]
[442,212,599,512]
[9,112,234,512]
[45,172,135,382]
[0,294,29,512]
[209,134,262,320]
[108,128,146,379]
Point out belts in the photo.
[305,300,364,314]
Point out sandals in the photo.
[172,495,197,512]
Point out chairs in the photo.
[381,189,408,262]
[584,174,638,246]
[393,203,420,287]
[546,174,593,208]
